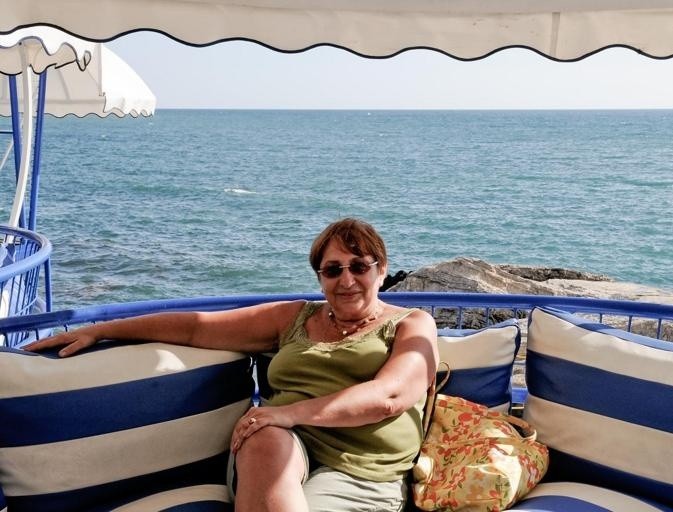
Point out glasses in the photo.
[314,260,382,279]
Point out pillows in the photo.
[0,241,30,349]
[257,314,522,434]
[522,302,672,504]
[1,335,254,512]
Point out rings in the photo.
[247,417,256,423]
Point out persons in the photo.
[18,219,440,511]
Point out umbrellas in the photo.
[2,26,158,170]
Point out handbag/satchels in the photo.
[411,361,551,511]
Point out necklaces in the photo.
[328,312,374,336]
